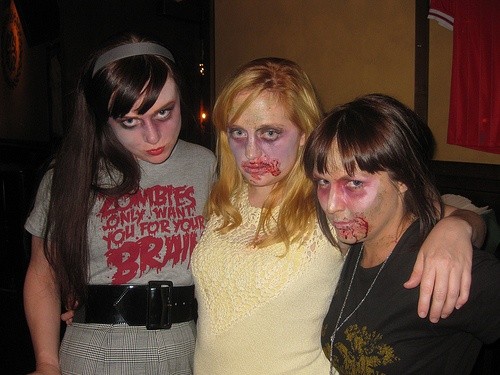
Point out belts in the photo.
[65,282,197,330]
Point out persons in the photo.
[302,95,500,375]
[59,56,487,375]
[22,35,217,375]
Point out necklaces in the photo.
[328,223,413,375]
